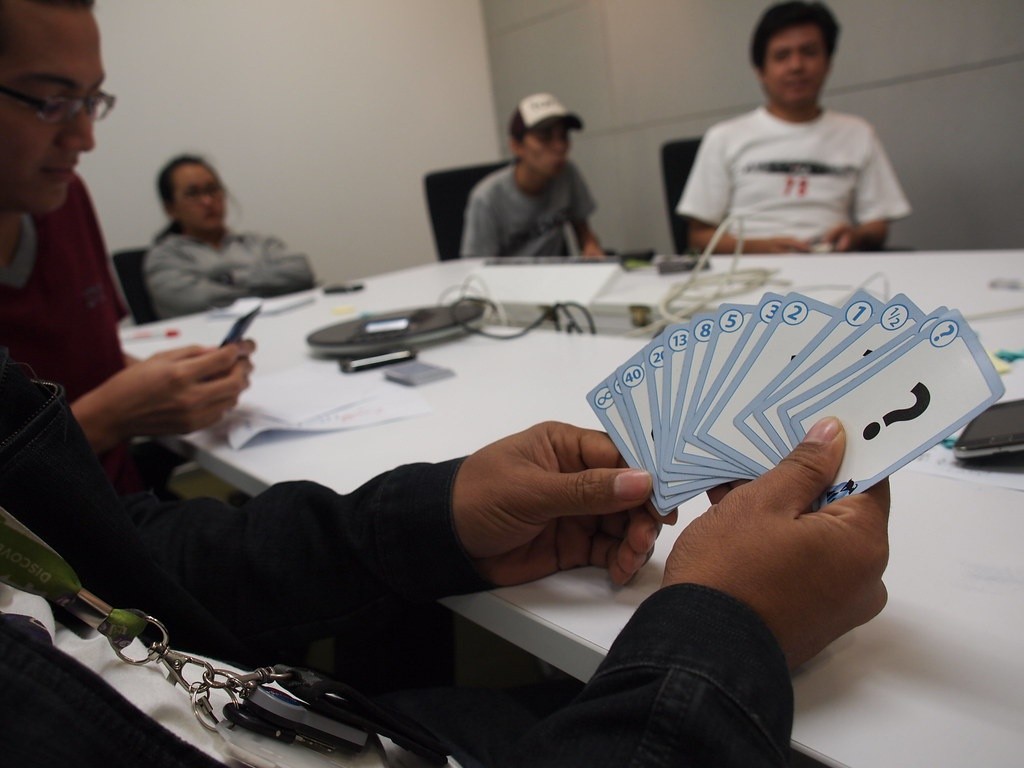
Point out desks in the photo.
[119,250,1024,767]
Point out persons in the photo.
[0,347,889,767]
[460,91,604,257]
[145,154,317,319]
[0,0,253,484]
[676,0,912,255]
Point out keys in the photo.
[0,509,448,767]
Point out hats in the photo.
[510,93,582,135]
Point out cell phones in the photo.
[954,398,1024,459]
[325,285,363,293]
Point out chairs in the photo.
[113,248,159,323]
[657,135,703,256]
[423,159,516,263]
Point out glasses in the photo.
[0,85,116,126]
